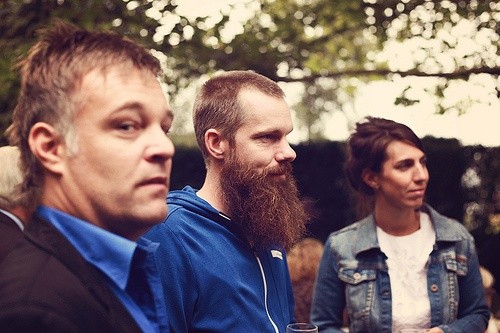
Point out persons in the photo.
[311,117,491,333]
[286,237,350,333]
[139,69,316,333]
[0,19,175,332]
[0,146,36,259]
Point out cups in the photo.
[286,323,318,333]
